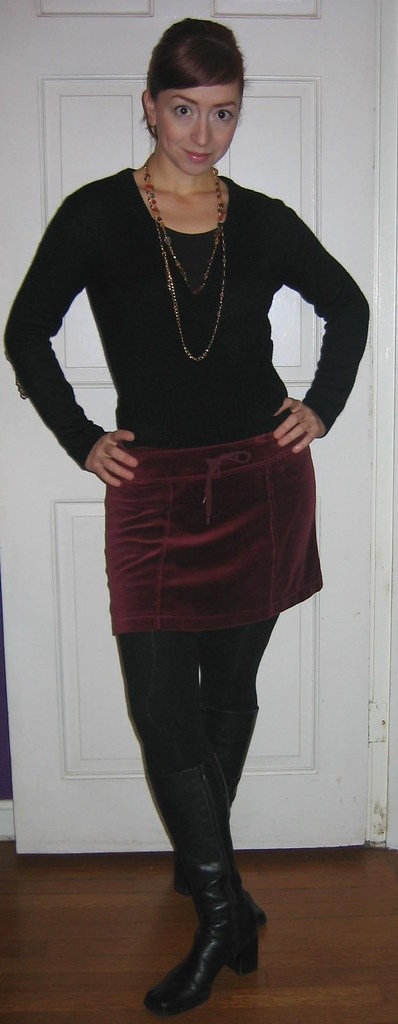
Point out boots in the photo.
[144,751,258,1018]
[173,701,268,927]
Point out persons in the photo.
[3,16,370,1013]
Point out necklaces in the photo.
[144,152,227,362]
